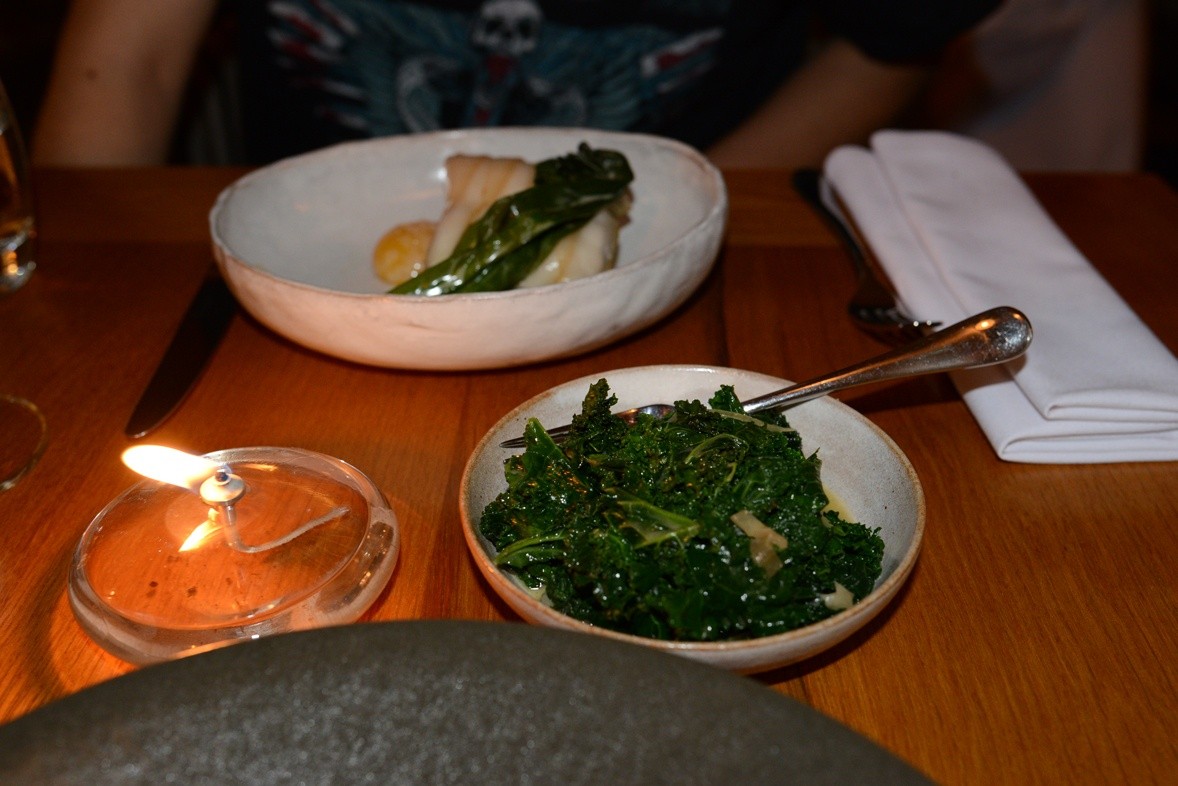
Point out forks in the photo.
[501,305,1034,471]
[789,167,942,332]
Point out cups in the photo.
[0,75,39,294]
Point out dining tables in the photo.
[0,164,1178,786]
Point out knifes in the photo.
[124,257,242,439]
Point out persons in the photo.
[34,2,1008,173]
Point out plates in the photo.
[209,126,726,368]
[456,365,925,675]
